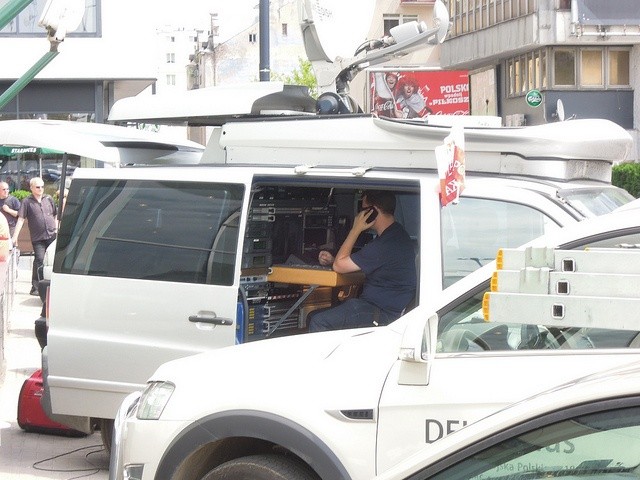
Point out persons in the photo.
[386,72,397,93]
[307,190,416,334]
[0,181,21,251]
[11,177,58,295]
[394,75,427,117]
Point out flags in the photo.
[435,120,466,208]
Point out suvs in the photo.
[107,200,640,480]
[44,164,79,175]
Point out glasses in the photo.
[36,186,44,188]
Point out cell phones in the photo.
[361,207,378,224]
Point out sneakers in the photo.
[30,286,40,295]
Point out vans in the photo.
[0,82,635,449]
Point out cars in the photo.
[27,169,70,185]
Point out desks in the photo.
[266,266,365,336]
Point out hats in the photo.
[398,77,418,92]
